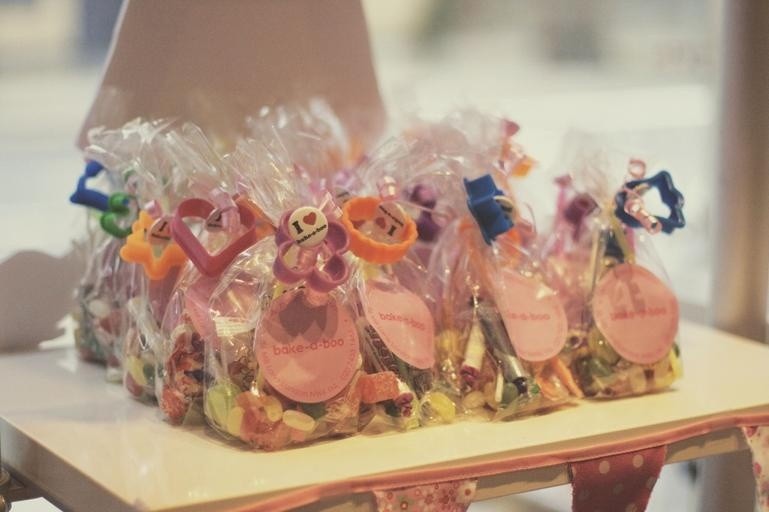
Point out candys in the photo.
[68,98,687,450]
